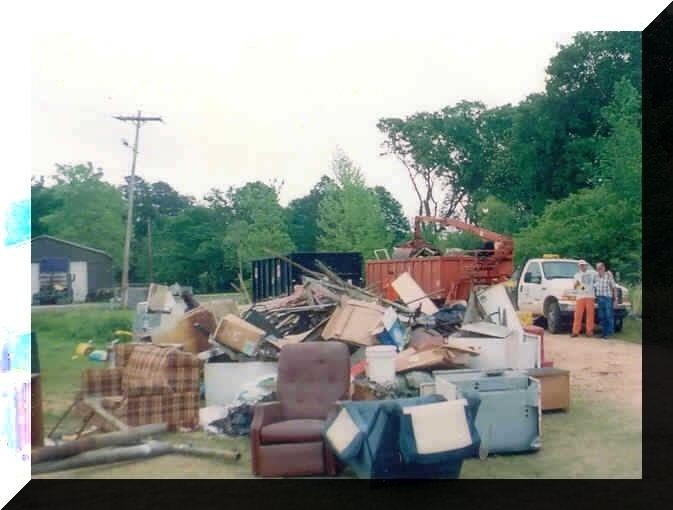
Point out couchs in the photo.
[73,340,203,436]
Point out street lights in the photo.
[120,139,139,312]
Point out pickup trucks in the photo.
[473,255,632,333]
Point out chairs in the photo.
[248,337,355,478]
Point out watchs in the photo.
[613,297,617,301]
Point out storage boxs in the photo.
[212,313,267,357]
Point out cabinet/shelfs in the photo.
[526,369,572,417]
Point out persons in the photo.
[569,259,613,338]
[593,262,619,339]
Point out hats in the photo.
[578,260,587,265]
